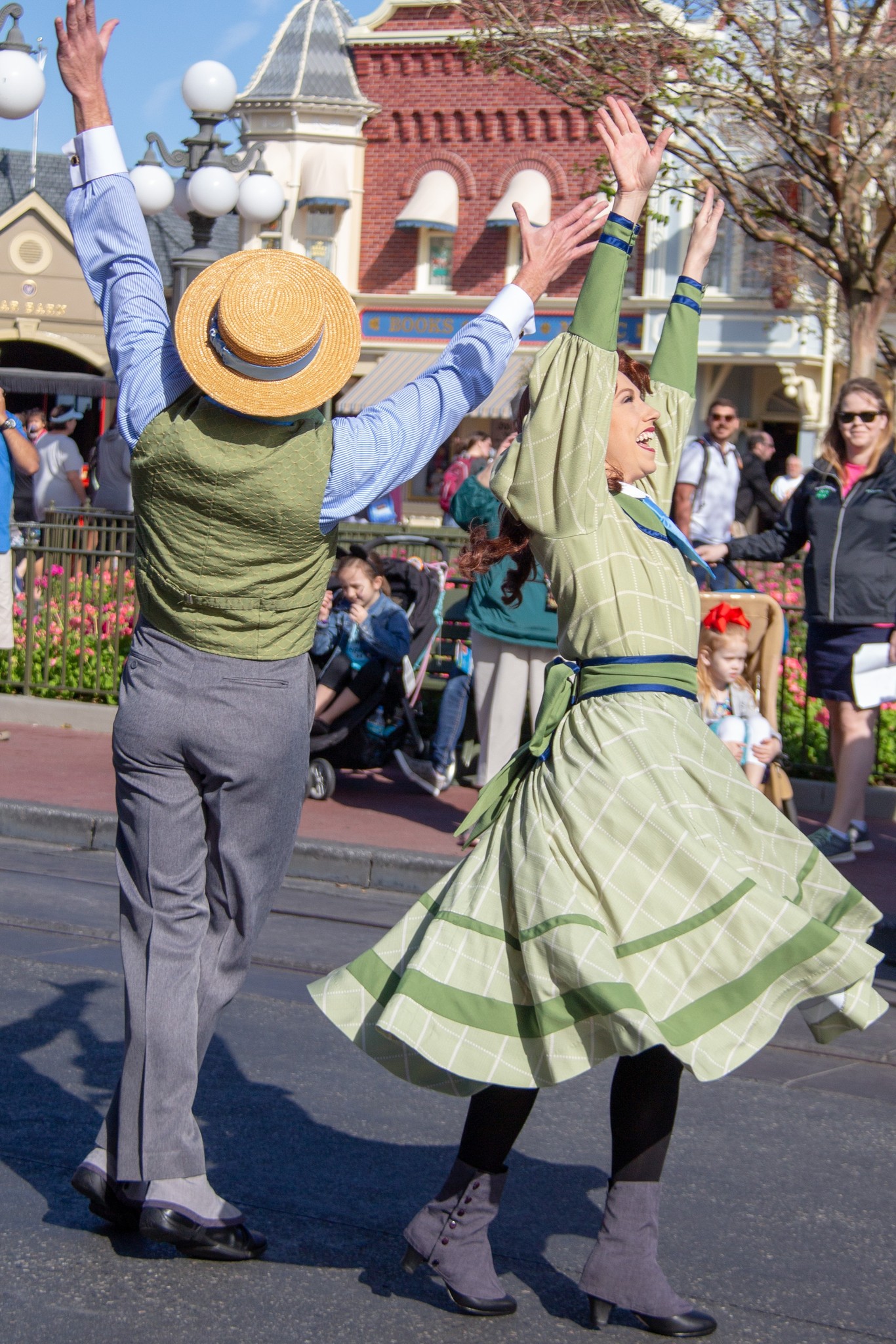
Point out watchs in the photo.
[0,417,16,432]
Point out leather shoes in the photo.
[72,1166,143,1223]
[140,1205,266,1260]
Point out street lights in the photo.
[124,59,288,328]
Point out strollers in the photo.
[692,541,803,833]
[308,535,450,800]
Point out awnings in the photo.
[298,153,350,210]
[486,169,552,227]
[587,191,615,228]
[336,351,534,419]
[395,170,459,232]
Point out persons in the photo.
[449,432,576,843]
[10,412,43,594]
[430,467,443,495]
[306,96,889,1338]
[672,377,896,863]
[34,404,98,575]
[440,430,492,526]
[87,405,136,575]
[394,676,475,794]
[310,543,412,733]
[52,0,609,1261]
[434,447,445,467]
[26,408,48,443]
[88,436,100,505]
[0,387,41,739]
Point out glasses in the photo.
[836,411,884,422]
[711,414,734,423]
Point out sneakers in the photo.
[394,748,446,796]
[805,823,875,863]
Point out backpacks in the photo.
[366,492,396,524]
[440,457,475,514]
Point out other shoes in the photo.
[12,569,24,595]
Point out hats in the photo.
[50,407,84,423]
[173,252,362,420]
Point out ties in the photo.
[638,496,717,581]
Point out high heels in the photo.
[403,1235,518,1315]
[590,1286,718,1338]
